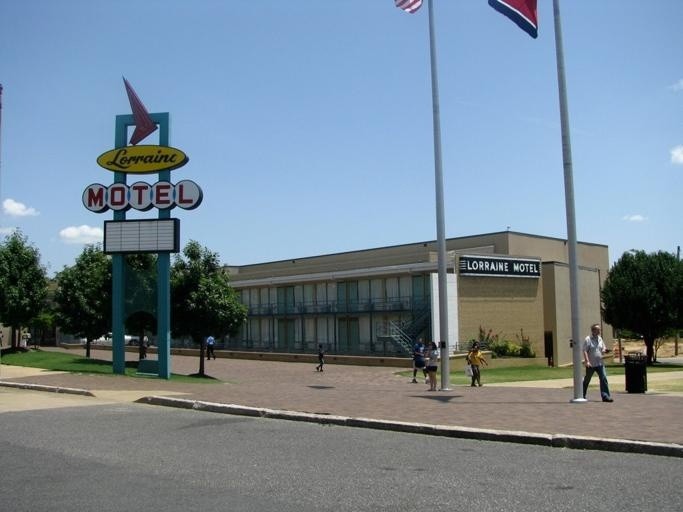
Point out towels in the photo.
[624,351,647,394]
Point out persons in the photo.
[581,323,614,402]
[465,341,484,368]
[0,330,5,347]
[467,346,487,387]
[410,338,438,391]
[142,334,149,359]
[205,335,216,360]
[315,343,326,372]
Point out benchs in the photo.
[391,0,424,15]
[485,0,538,39]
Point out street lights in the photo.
[465,359,474,378]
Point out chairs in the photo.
[141,355,148,360]
[602,398,613,403]
[472,384,483,387]
[411,378,439,391]
[207,355,217,360]
[316,367,323,372]
